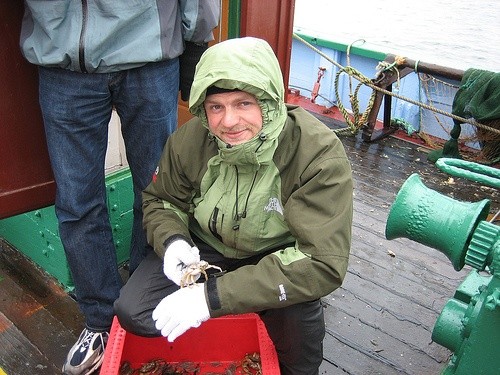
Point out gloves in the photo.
[151,239,211,342]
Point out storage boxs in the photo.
[99,313,280,375]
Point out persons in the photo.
[113,36,353,375]
[20,0,220,375]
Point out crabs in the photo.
[180,260,222,290]
[121,353,260,375]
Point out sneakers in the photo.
[61,327,110,375]
